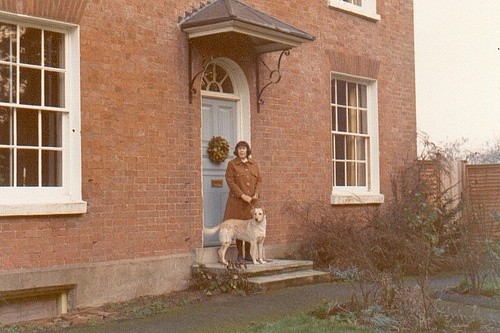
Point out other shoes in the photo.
[237,256,253,264]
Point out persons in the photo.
[222,140,264,264]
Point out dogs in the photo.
[203,205,270,264]
